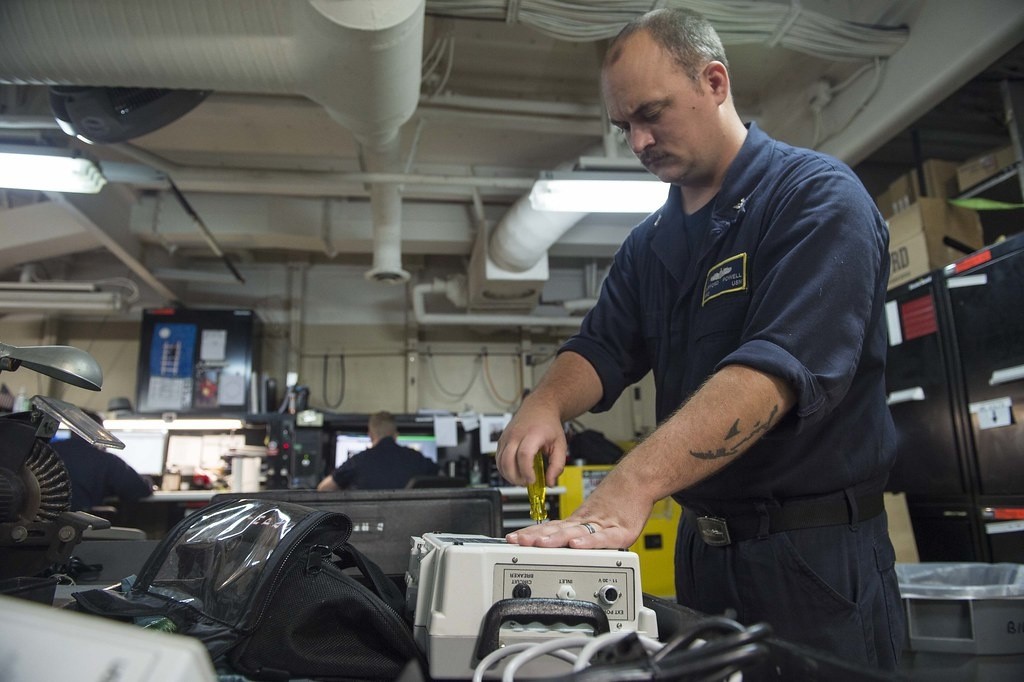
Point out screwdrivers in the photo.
[526,451,547,524]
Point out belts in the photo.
[679,480,884,550]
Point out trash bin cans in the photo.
[894,560,1024,682]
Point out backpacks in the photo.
[61,499,415,682]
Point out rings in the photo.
[579,522,596,534]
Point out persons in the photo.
[494,9,912,682]
[317,410,448,492]
[48,407,152,513]
